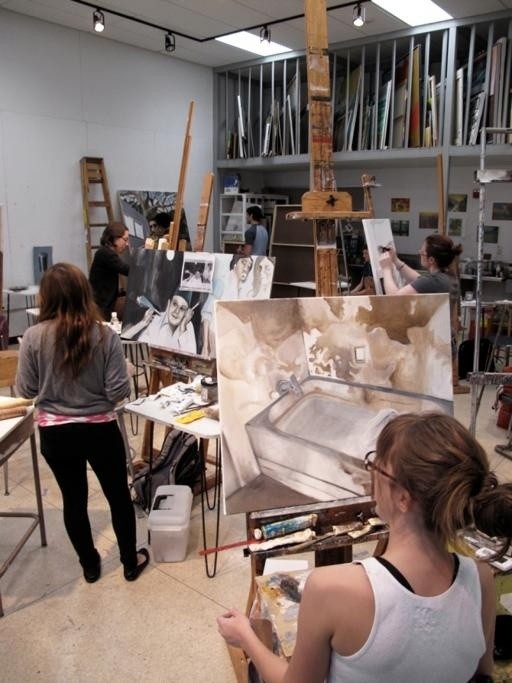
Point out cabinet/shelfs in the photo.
[218,193,290,244]
[269,202,316,298]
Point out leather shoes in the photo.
[123,547,150,582]
[79,549,101,583]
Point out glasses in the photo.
[418,252,429,258]
[362,447,416,501]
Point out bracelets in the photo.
[397,262,405,270]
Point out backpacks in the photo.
[130,429,205,515]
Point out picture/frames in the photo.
[381,43,424,147]
[435,67,463,146]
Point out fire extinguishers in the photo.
[493,365,512,430]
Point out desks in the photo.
[1,396,46,617]
[3,282,41,342]
[124,373,222,578]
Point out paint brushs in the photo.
[287,522,363,552]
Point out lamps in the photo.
[165,32,176,51]
[352,5,366,27]
[92,10,105,33]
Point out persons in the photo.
[201,254,253,360]
[237,205,270,256]
[378,234,463,362]
[120,308,154,340]
[183,271,202,289]
[343,244,376,295]
[138,284,197,357]
[15,263,150,583]
[216,412,512,683]
[154,212,178,251]
[87,222,130,322]
[248,255,276,299]
[148,218,163,250]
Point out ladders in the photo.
[77,155,116,270]
[464,125,511,439]
